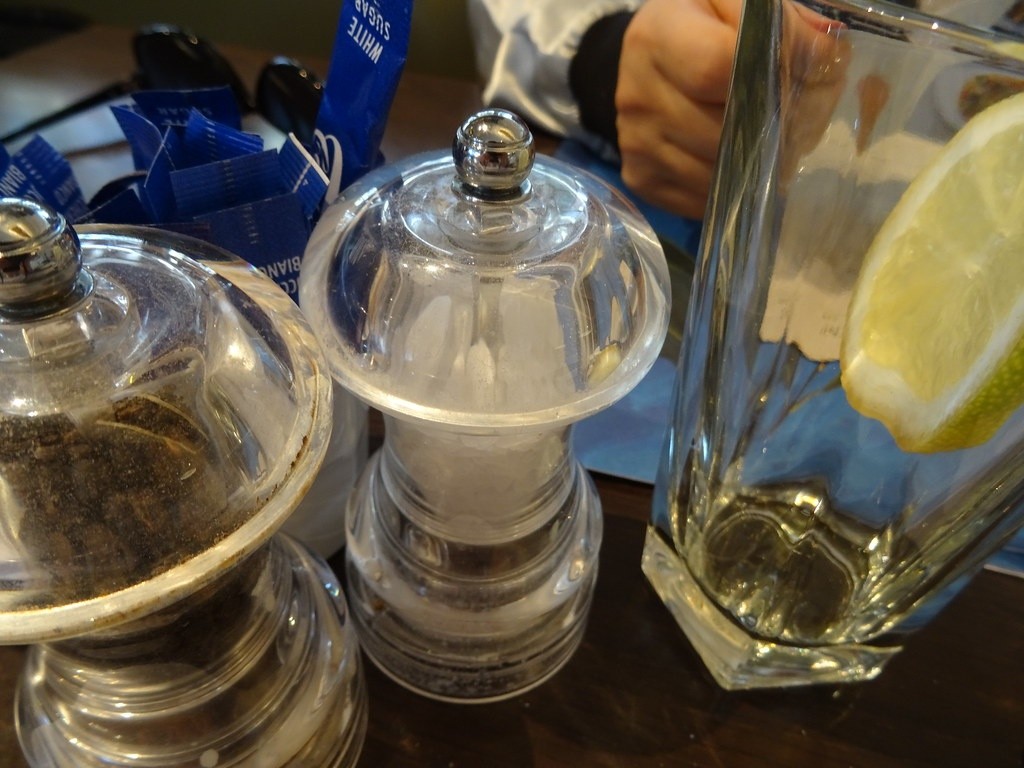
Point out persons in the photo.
[469,0,1024,576]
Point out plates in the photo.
[931,60,1024,134]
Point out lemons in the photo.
[839,91,1023,455]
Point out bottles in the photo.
[0,102,670,768]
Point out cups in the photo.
[640,0,1024,689]
